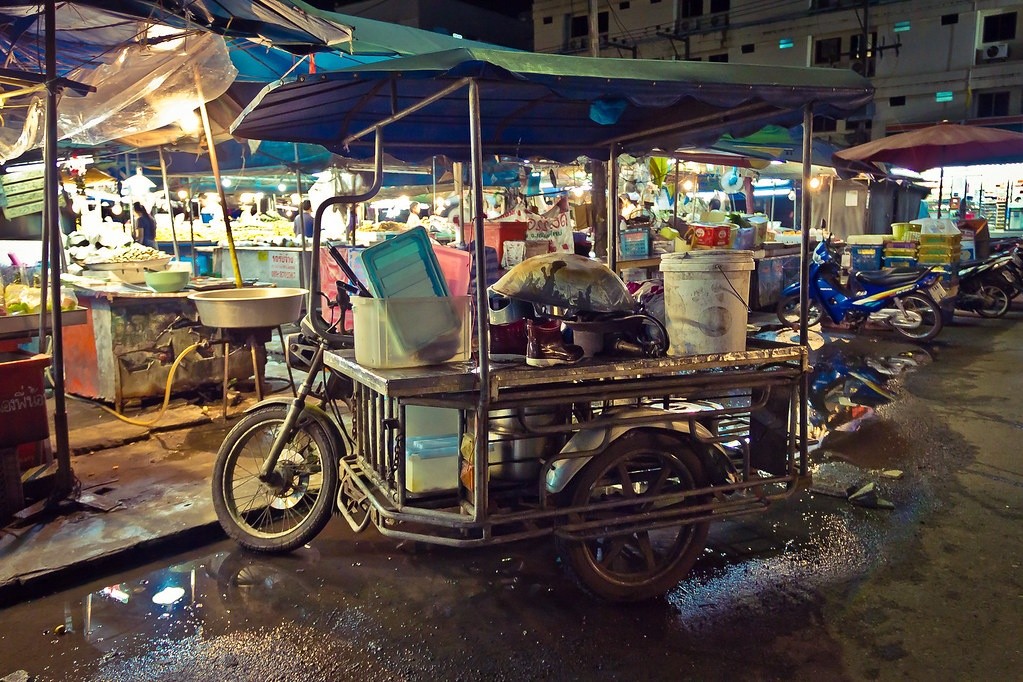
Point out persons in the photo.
[185,193,207,222]
[133,201,159,249]
[198,193,214,223]
[620,193,636,217]
[708,199,730,223]
[405,202,421,227]
[295,199,313,237]
[488,205,501,218]
[619,198,627,229]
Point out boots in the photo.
[489,319,528,362]
[524,321,584,366]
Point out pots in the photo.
[563,314,649,358]
[491,253,669,355]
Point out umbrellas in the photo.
[831,120,1023,219]
[717,123,888,214]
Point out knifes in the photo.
[226,278,259,283]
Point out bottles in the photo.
[620,219,627,231]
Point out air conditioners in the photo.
[848,59,870,76]
[566,37,583,50]
[678,12,728,32]
[982,42,1009,61]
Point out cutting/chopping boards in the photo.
[187,278,252,291]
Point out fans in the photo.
[718,166,744,194]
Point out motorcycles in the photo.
[752,323,896,428]
[776,219,1023,344]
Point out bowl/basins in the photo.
[188,288,309,328]
[73,254,176,283]
[144,271,188,292]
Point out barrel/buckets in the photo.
[661,250,755,355]
[891,223,921,242]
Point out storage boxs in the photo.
[0,349,54,448]
[844,216,993,271]
[458,199,768,271]
[347,226,474,368]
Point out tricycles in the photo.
[211,47,877,608]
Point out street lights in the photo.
[178,191,187,220]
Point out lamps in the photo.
[120,140,158,191]
